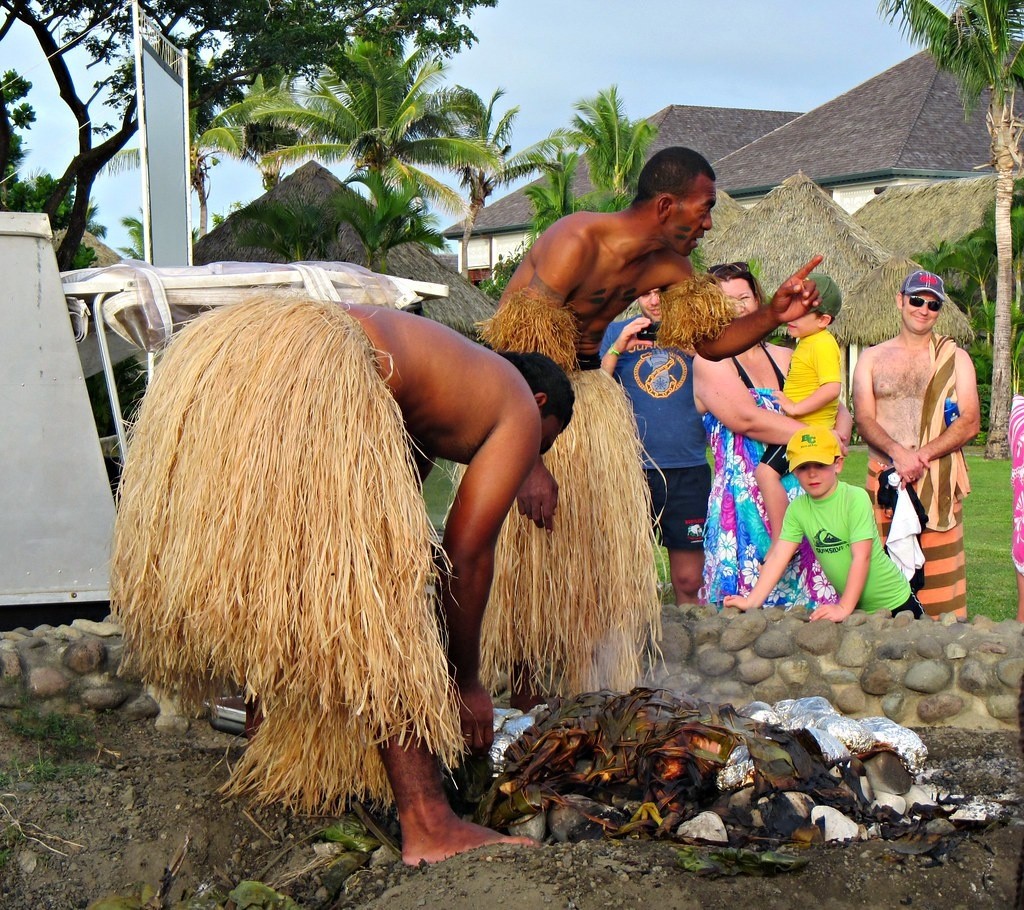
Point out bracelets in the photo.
[609,344,619,356]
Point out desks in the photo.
[60,268,449,474]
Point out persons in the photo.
[690,263,853,611]
[113,300,578,866]
[444,145,824,709]
[852,270,980,623]
[753,273,842,571]
[723,425,925,623]
[599,288,712,607]
[1010,393,1024,625]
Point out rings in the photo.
[462,733,473,737]
[910,476,913,479]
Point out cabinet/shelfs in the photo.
[0,211,120,606]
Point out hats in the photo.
[900,271,945,302]
[786,427,842,473]
[801,273,842,316]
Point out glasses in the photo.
[706,262,751,273]
[908,295,941,311]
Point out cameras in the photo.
[637,322,662,341]
